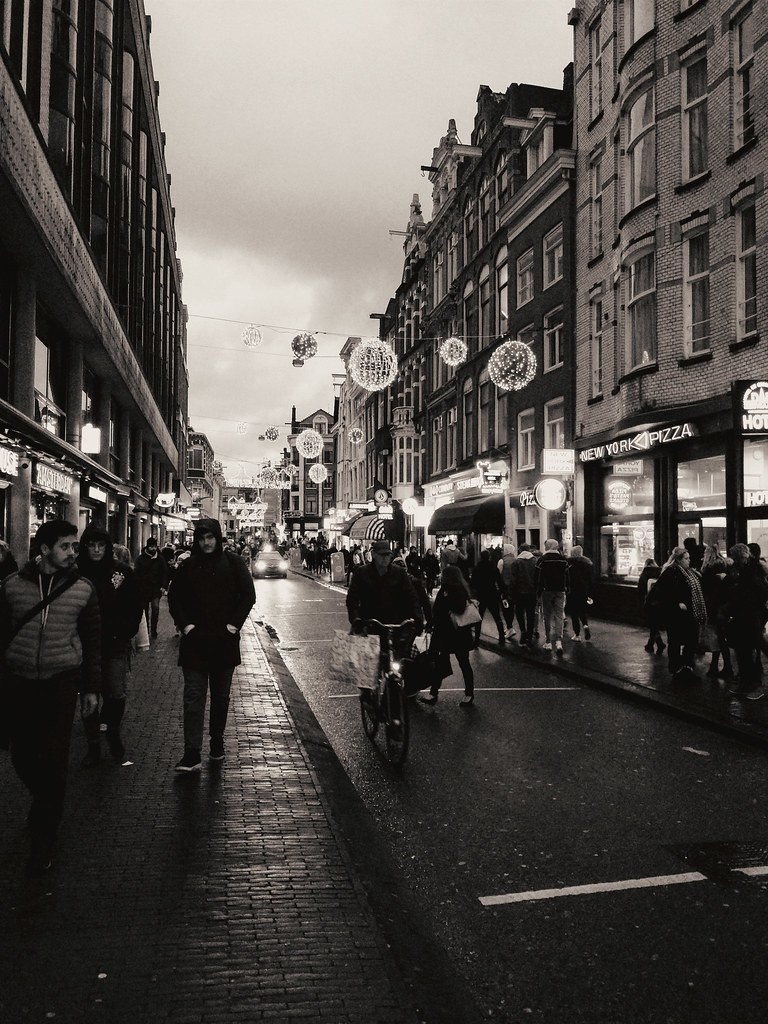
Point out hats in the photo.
[373,541,394,555]
[147,538,157,546]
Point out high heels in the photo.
[419,696,437,705]
[459,696,474,705]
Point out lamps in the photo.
[237,325,538,484]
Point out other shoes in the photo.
[644,642,764,700]
[81,747,101,770]
[26,846,51,879]
[106,731,124,759]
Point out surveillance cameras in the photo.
[20,457,30,469]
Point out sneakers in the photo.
[209,737,225,759]
[474,627,591,651]
[174,753,201,771]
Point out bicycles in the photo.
[358,618,426,765]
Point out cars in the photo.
[251,551,287,579]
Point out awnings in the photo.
[349,514,398,540]
[428,495,505,535]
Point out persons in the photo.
[223,536,261,570]
[168,519,256,771]
[637,536,768,700]
[135,538,191,638]
[0,520,145,872]
[278,535,349,575]
[346,541,423,702]
[392,558,431,698]
[393,539,593,655]
[415,565,475,707]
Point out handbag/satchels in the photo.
[328,623,380,690]
[414,632,452,690]
[698,623,720,652]
[445,589,482,631]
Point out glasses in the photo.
[85,543,106,550]
